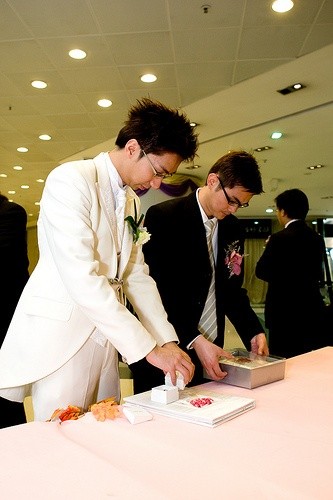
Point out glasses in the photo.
[217,176,249,209]
[141,147,172,179]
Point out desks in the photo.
[0,346,333,500]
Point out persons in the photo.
[1,96,199,429]
[117,151,269,396]
[0,194,29,429]
[255,189,329,357]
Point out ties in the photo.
[198,220,218,344]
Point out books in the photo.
[121,384,255,429]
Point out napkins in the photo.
[123,406,152,424]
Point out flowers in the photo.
[224,240,249,279]
[124,213,152,248]
[88,396,119,422]
[45,404,85,426]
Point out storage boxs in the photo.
[203,348,285,390]
